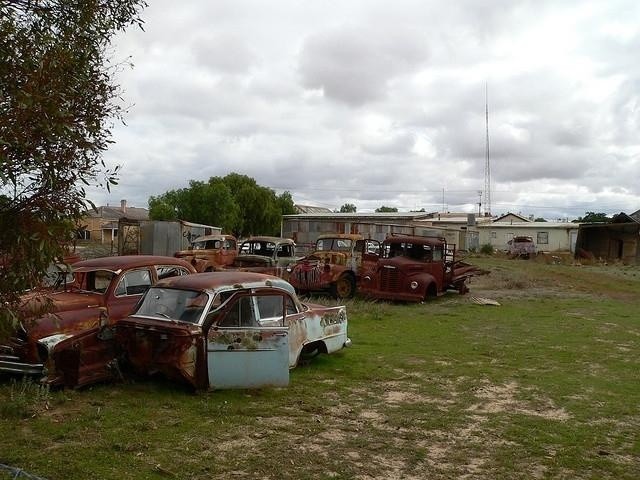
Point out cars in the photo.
[237,242,375,250]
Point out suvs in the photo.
[0,255,201,384]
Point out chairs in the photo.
[412,244,424,257]
[389,243,403,258]
[179,308,204,324]
